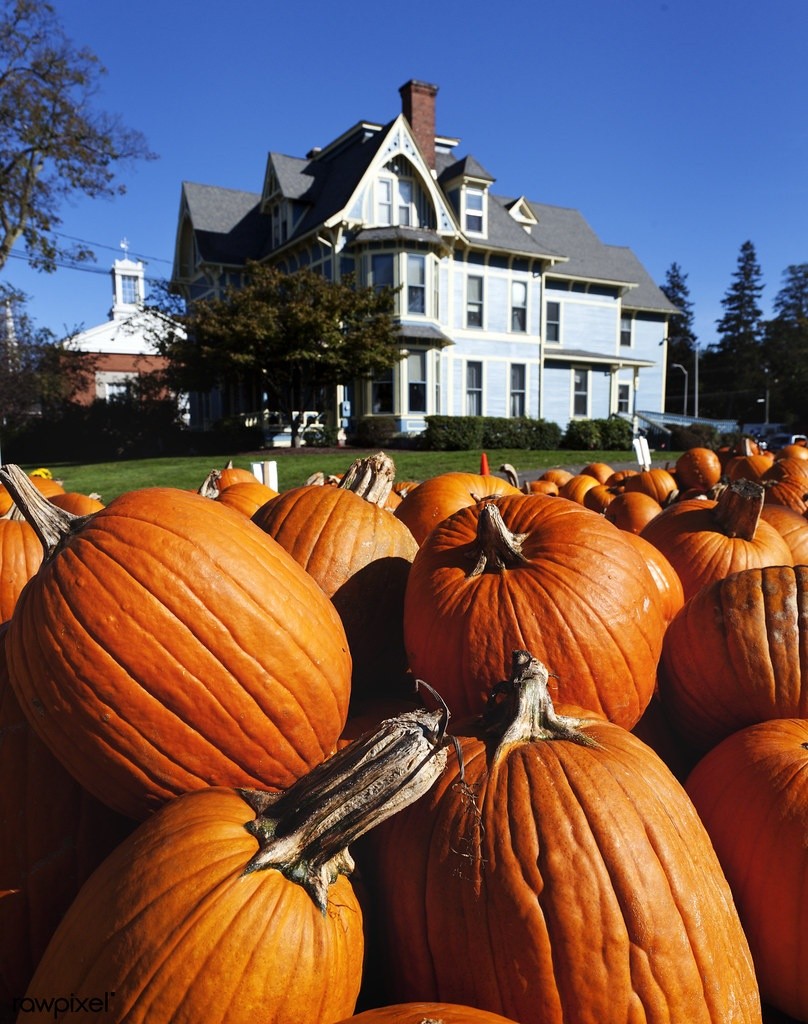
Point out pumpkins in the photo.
[0,440,808,1024]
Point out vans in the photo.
[744,423,786,450]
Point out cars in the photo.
[768,434,807,453]
